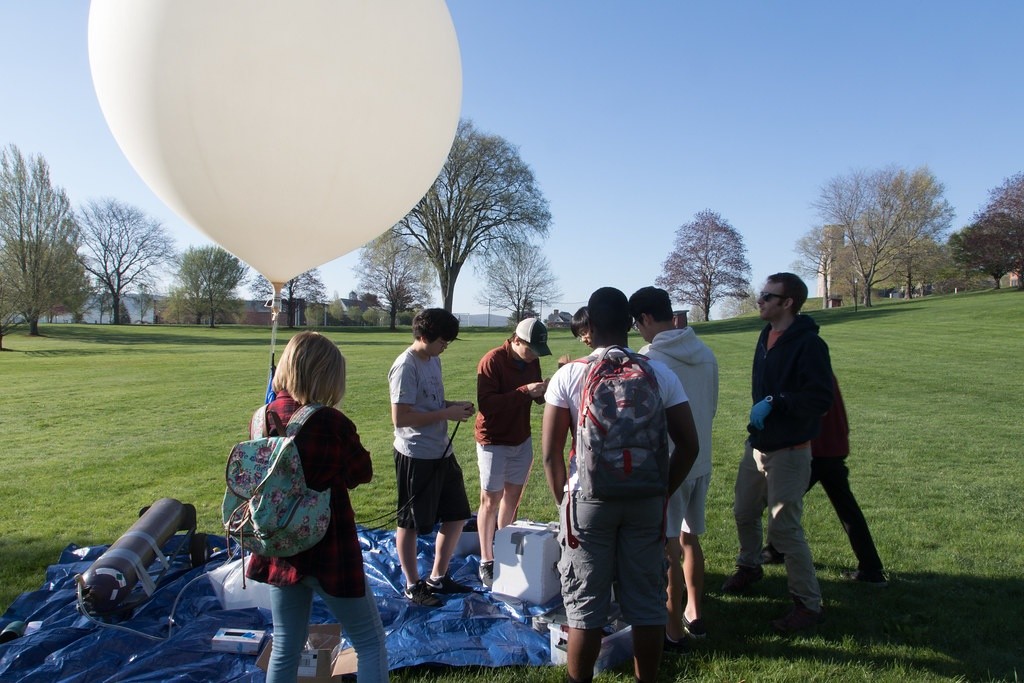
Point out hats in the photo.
[515,318,552,357]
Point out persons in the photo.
[763,379,885,583]
[557,307,591,368]
[543,286,700,683]
[629,286,719,645]
[388,307,475,609]
[721,271,836,632]
[245,330,390,683]
[474,318,550,588]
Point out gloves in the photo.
[750,400,773,430]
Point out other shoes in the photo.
[850,565,886,584]
[681,610,706,638]
[666,624,685,643]
[760,546,785,565]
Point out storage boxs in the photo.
[453,532,481,556]
[255,623,359,683]
[212,627,266,652]
[492,520,565,603]
[548,616,634,678]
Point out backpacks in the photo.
[222,403,334,558]
[572,345,669,498]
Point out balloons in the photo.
[88,0,461,315]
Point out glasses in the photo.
[760,291,788,302]
[632,314,643,331]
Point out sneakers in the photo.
[721,564,764,593]
[425,572,474,594]
[404,579,442,606]
[773,596,825,633]
[478,560,493,588]
